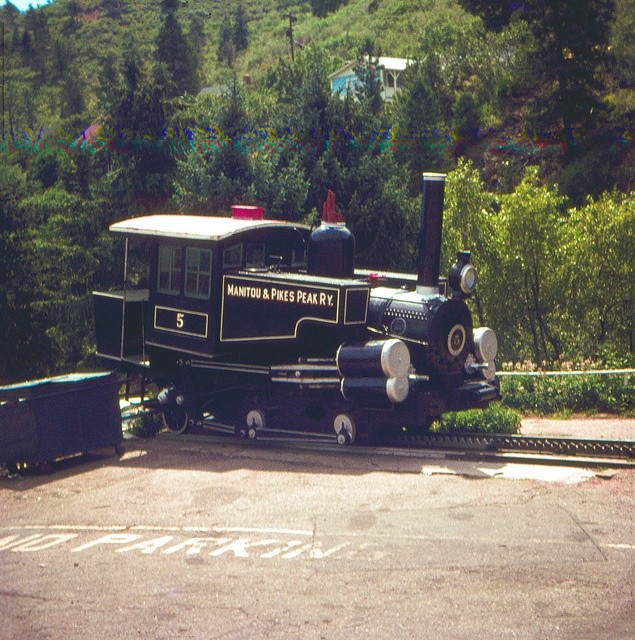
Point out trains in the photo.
[90,171,504,444]
